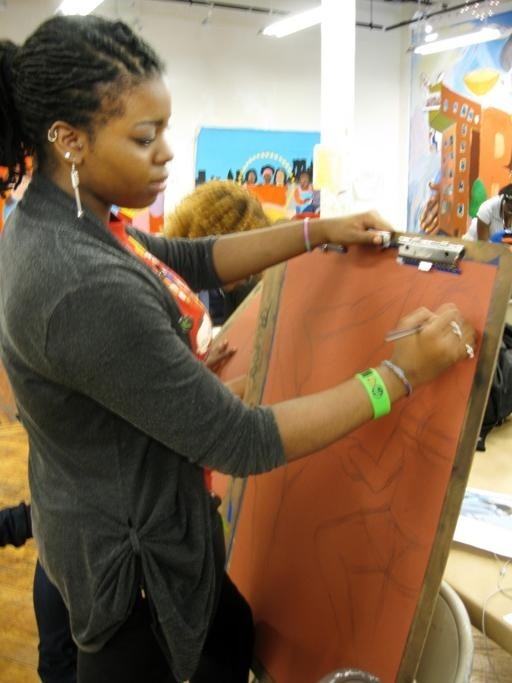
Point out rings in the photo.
[449,319,462,340]
[465,343,475,359]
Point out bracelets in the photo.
[354,366,393,421]
[302,215,315,254]
[379,358,413,398]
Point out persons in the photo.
[151,180,276,327]
[0,11,480,683]
[460,182,511,243]
[487,201,512,246]
[239,165,319,216]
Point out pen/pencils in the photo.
[384,324,423,343]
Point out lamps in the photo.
[54,0,103,18]
[405,1,512,56]
[259,2,324,39]
